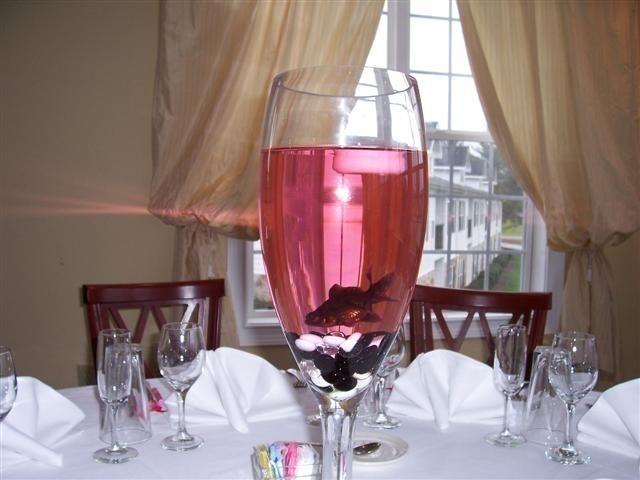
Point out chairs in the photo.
[81,276,227,387]
[407,282,554,399]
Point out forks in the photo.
[286,368,308,388]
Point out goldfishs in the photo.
[304,264,400,328]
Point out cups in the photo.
[1,346,18,425]
[522,345,573,448]
[98,342,153,447]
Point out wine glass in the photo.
[483,323,528,448]
[362,322,406,430]
[91,328,139,464]
[255,64,431,480]
[156,321,206,453]
[544,330,601,466]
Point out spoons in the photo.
[280,439,382,456]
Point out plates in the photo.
[353,431,409,463]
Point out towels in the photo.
[1,370,86,469]
[161,345,307,434]
[573,370,640,457]
[375,347,516,428]
